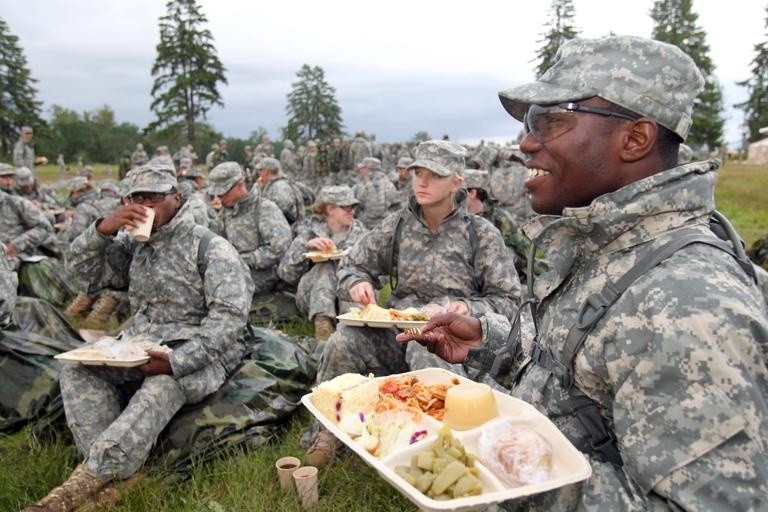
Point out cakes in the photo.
[311,373,380,421]
[362,304,389,320]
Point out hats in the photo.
[406,139,467,177]
[101,182,120,195]
[15,166,35,186]
[66,177,89,199]
[208,161,243,196]
[123,164,179,199]
[315,184,361,207]
[497,33,707,144]
[473,145,527,168]
[136,130,382,170]
[464,169,489,190]
[20,126,34,135]
[0,162,17,177]
[395,157,414,170]
[185,167,206,179]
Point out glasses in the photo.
[522,102,638,144]
[129,190,178,204]
[339,205,358,213]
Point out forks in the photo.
[405,326,423,340]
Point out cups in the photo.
[274,456,320,511]
[129,206,155,243]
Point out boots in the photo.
[73,470,150,512]
[83,294,120,325]
[63,290,94,321]
[19,462,106,512]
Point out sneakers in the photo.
[303,429,346,469]
[313,314,335,340]
